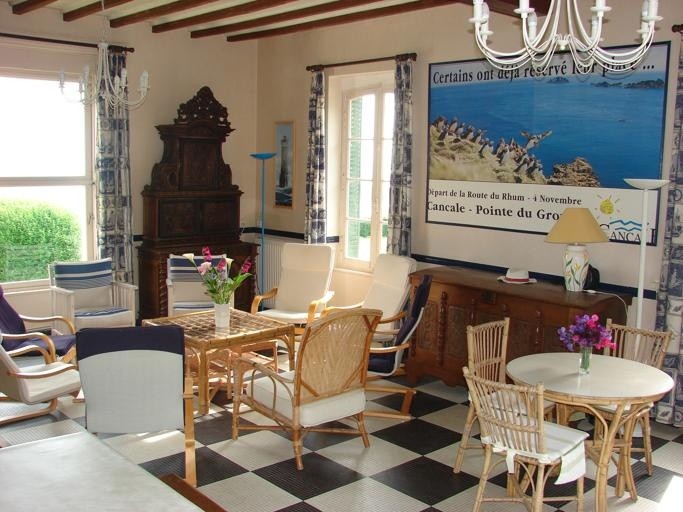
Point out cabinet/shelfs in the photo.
[142,195,240,241]
[135,245,261,319]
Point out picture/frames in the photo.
[273,120,296,209]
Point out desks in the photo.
[506,352,675,510]
[404,263,630,393]
[142,307,298,413]
[0,429,202,512]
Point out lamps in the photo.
[543,209,610,292]
[58,6,151,116]
[468,1,662,76]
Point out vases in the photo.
[578,345,592,375]
[211,302,231,329]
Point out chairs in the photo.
[49,258,138,331]
[250,238,336,322]
[165,253,238,314]
[1,344,80,418]
[461,363,588,511]
[320,253,432,380]
[1,287,76,362]
[602,317,670,476]
[231,310,384,470]
[452,315,517,472]
[75,326,202,485]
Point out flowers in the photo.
[555,314,619,351]
[181,249,262,304]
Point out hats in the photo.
[497,268,537,284]
